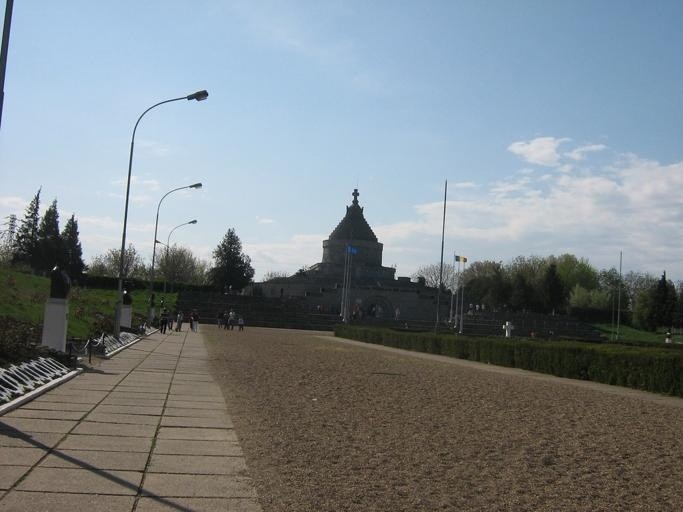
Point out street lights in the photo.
[111,90,209,340]
[149,183,202,300]
[162,220,196,298]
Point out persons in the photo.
[664,328,672,348]
